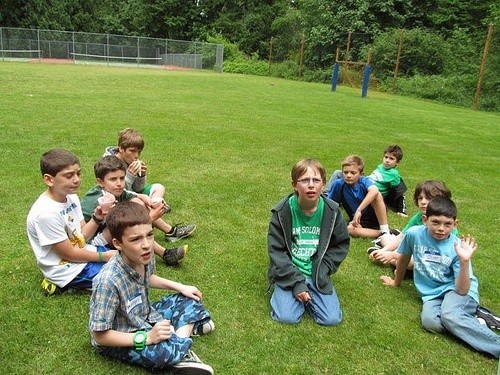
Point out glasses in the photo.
[296,178,323,186]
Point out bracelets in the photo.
[98,251,104,263]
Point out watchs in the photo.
[133,330,147,350]
[92,214,104,225]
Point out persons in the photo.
[89,201,216,375]
[26,127,196,296]
[326,144,500,359]
[264,157,350,326]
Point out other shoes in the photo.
[41,278,61,296]
[164,203,171,213]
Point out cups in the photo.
[98,195,115,214]
[150,198,163,212]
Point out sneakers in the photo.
[163,245,189,265]
[367,228,401,263]
[165,224,196,243]
[475,305,500,330]
[191,319,215,337]
[163,349,214,375]
